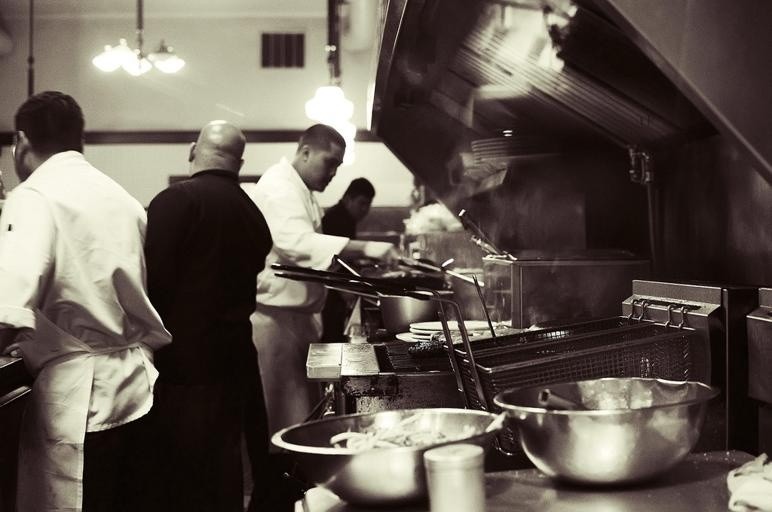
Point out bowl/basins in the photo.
[272,403,503,506]
[491,374,724,486]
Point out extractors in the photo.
[365,0,772,177]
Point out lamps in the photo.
[87,1,188,76]
[304,44,355,125]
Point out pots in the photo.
[272,254,486,333]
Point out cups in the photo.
[424,444,484,512]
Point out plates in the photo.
[471,130,567,168]
[395,320,497,343]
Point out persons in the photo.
[144,119,274,512]
[0,91,173,512]
[248,124,398,453]
[320,177,375,343]
